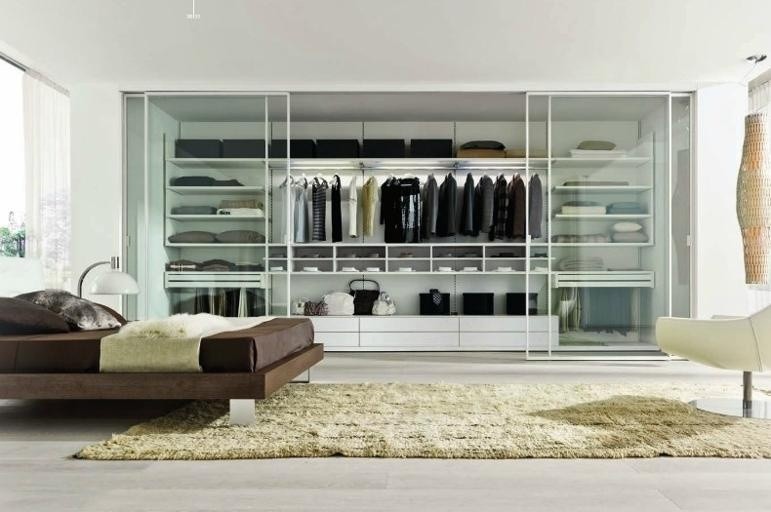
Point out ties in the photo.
[430,289,441,306]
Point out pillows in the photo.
[216,230,265,242]
[578,136,616,152]
[462,137,508,154]
[167,231,216,243]
[28,285,121,332]
[0,295,70,332]
[612,230,649,244]
[611,220,643,231]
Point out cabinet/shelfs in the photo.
[122,91,693,353]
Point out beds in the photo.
[1,284,328,424]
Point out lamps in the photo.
[77,253,139,304]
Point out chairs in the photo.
[654,280,770,423]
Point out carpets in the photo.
[70,373,770,460]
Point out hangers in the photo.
[278,169,542,186]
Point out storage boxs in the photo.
[418,290,540,314]
[174,139,456,161]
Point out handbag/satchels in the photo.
[291,300,328,316]
[351,290,380,315]
[323,293,355,316]
[372,300,396,315]
